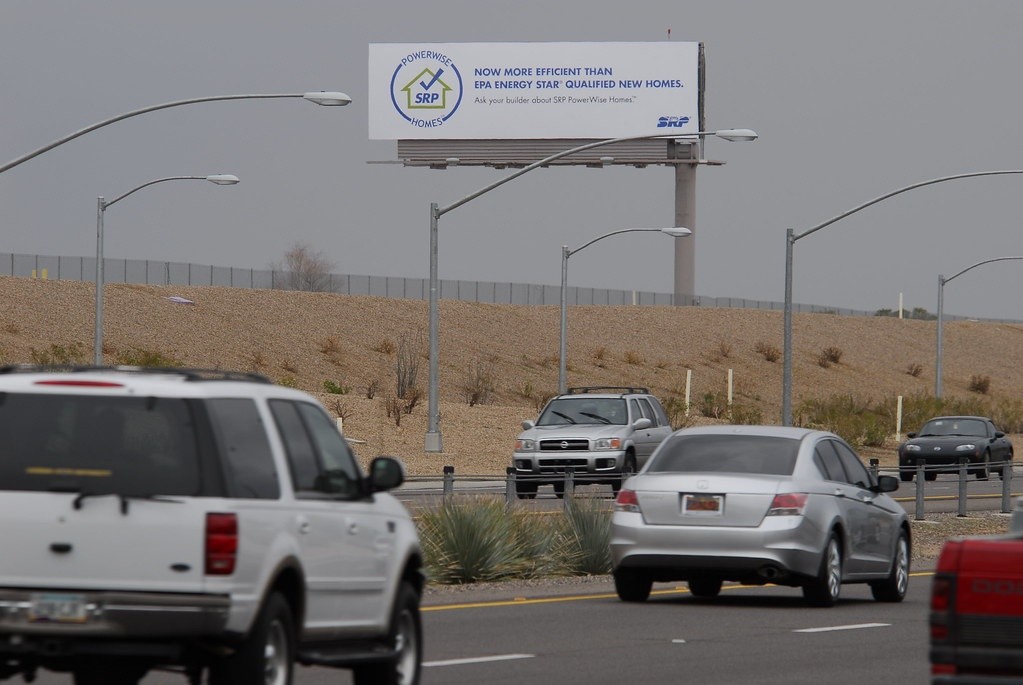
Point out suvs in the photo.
[512,386,674,500]
[1,362,427,685]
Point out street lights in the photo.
[426,128,758,455]
[95,175,239,364]
[557,226,694,391]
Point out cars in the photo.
[608,424,914,607]
[898,415,1013,481]
